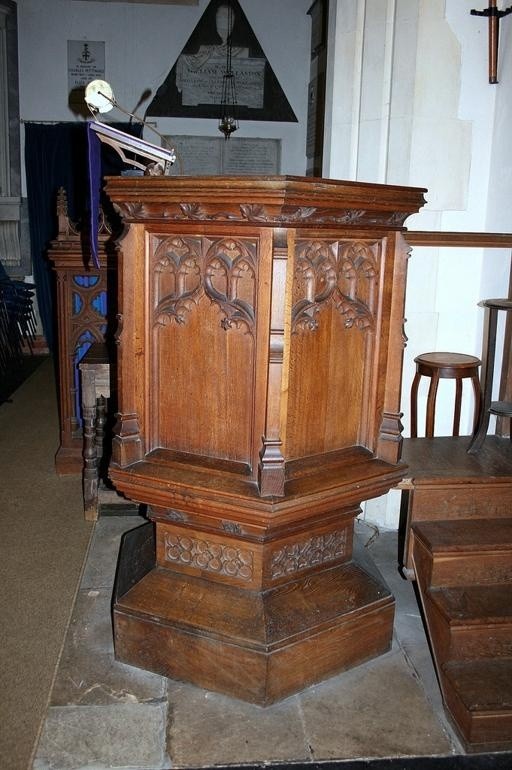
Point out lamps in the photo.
[218,0,244,148]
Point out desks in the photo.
[70,335,145,522]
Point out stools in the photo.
[408,348,486,444]
[467,294,512,456]
